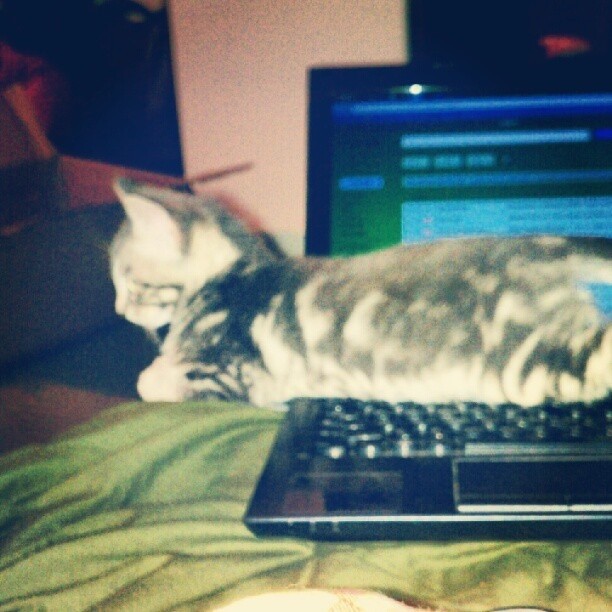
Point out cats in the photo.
[106,176,611,413]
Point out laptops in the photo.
[241,52,612,540]
